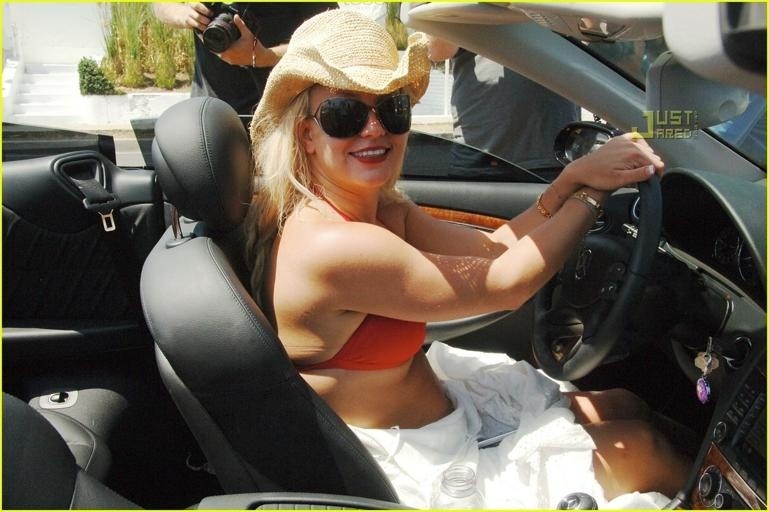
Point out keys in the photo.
[709,336,741,361]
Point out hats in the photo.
[248,8,431,152]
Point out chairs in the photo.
[139,96,413,511]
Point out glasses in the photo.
[305,92,412,139]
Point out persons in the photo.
[256,9,693,507]
[407,3,582,184]
[150,4,341,119]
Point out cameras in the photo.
[193,3,241,53]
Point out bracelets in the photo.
[535,191,550,220]
[564,189,603,224]
